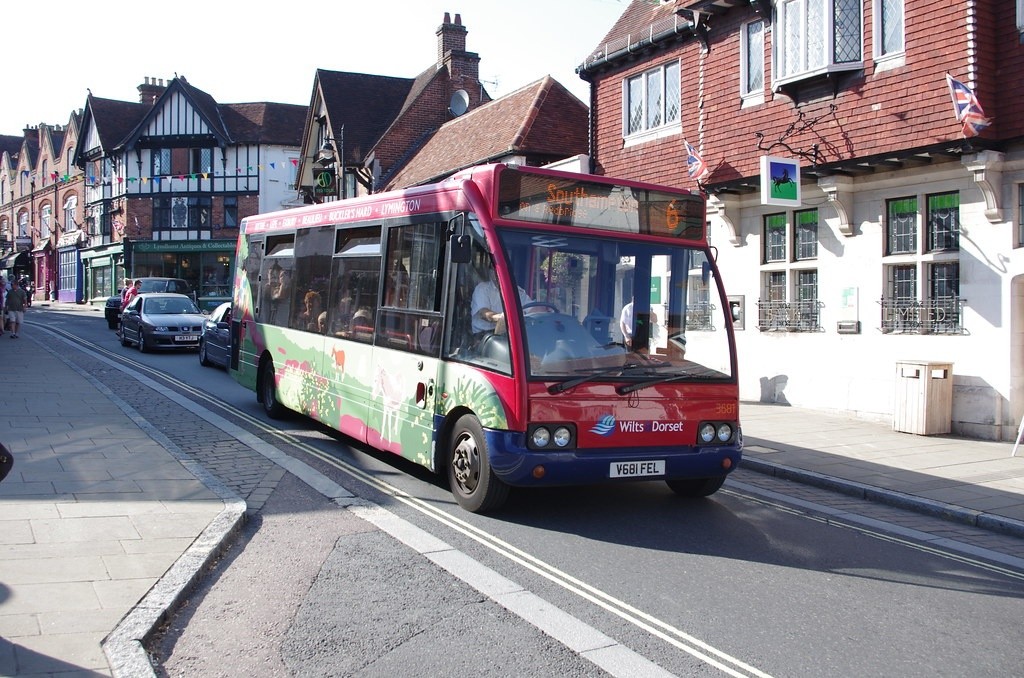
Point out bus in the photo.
[230,163,744,513]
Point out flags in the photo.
[946,73,995,139]
[682,140,709,180]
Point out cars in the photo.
[199,301,231,373]
[105,278,200,329]
[119,293,207,354]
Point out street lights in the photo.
[319,124,346,200]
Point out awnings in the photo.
[153,196,170,210]
[223,197,237,207]
[199,197,212,205]
[55,231,81,248]
[5,252,29,269]
[31,238,51,254]
[187,197,198,205]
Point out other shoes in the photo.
[116,331,120,336]
[10,334,19,339]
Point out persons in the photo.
[620,296,657,352]
[334,254,409,340]
[115,280,142,338]
[264,267,295,326]
[471,257,550,358]
[0,270,29,339]
[296,289,327,335]
[121,280,133,305]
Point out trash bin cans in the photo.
[892,359,955,436]
